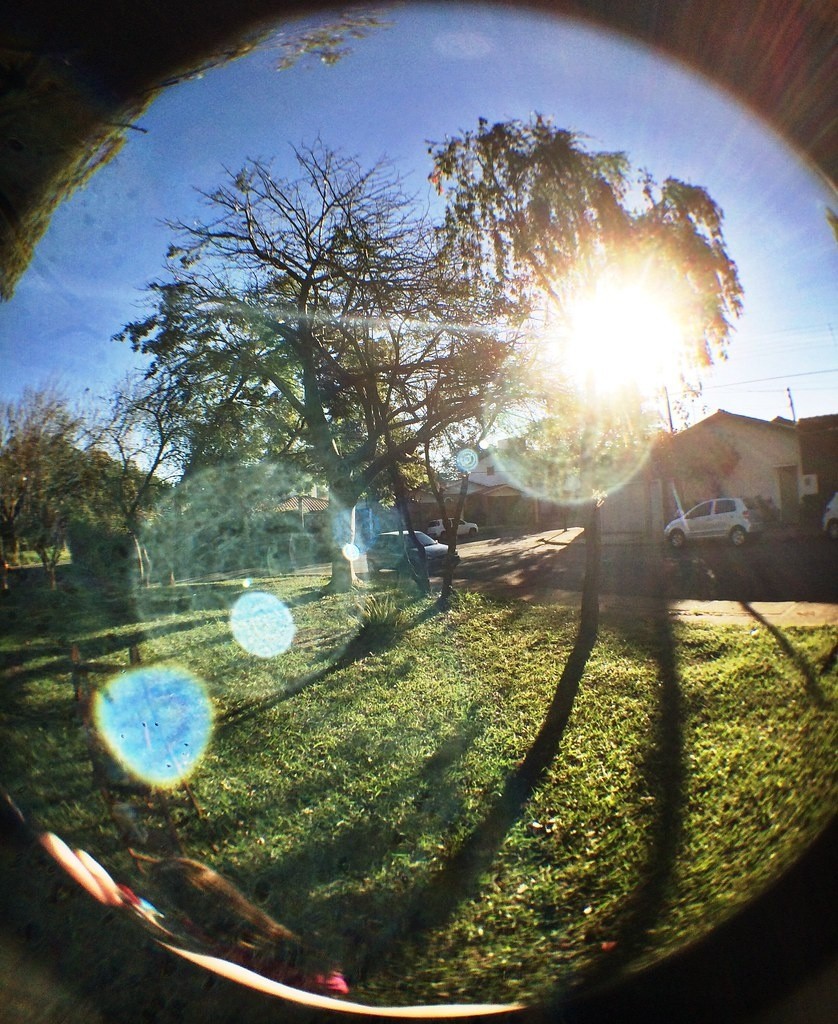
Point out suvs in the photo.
[429,519,478,541]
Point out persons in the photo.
[141,549,153,589]
[672,504,681,521]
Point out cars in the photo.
[366,531,462,577]
[822,490,838,539]
[662,494,764,549]
